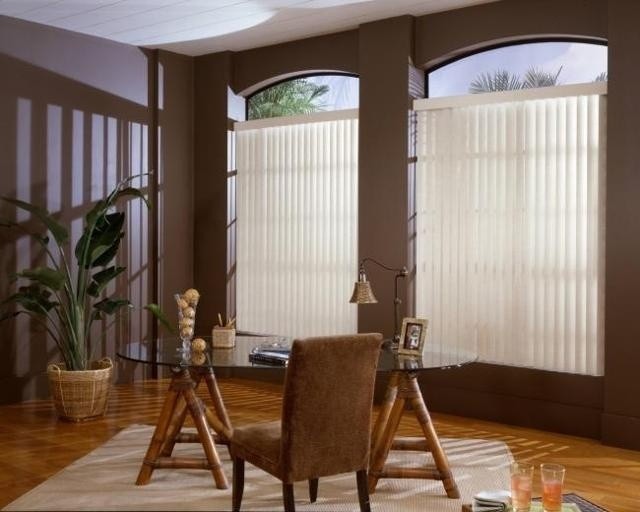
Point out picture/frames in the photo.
[397,318,429,356]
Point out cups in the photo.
[510,462,535,512]
[540,462,566,512]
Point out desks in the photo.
[115,336,479,499]
[462,491,610,512]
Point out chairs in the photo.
[230,332,385,512]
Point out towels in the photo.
[472,490,510,512]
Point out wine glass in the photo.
[174,294,200,353]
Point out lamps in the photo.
[349,258,408,350]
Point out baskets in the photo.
[48,357,112,423]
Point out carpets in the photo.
[0,423,514,512]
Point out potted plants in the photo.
[0,168,181,423]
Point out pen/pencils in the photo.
[226,316,236,328]
[217,311,223,327]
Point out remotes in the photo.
[250,352,286,366]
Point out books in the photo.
[249,351,290,368]
